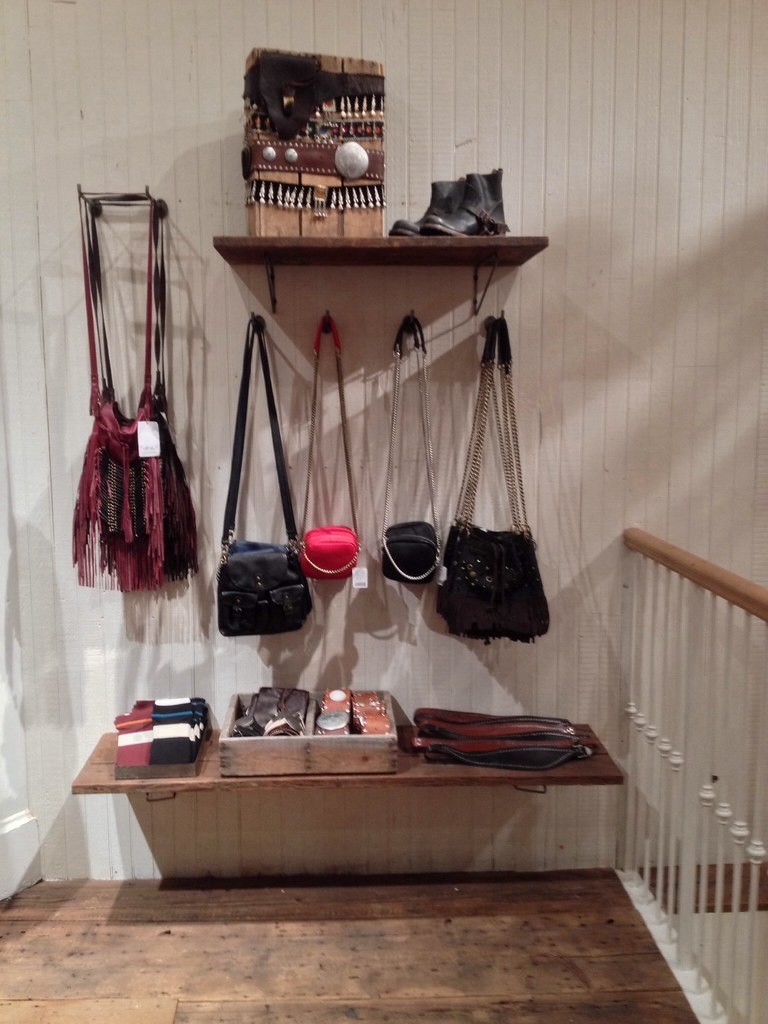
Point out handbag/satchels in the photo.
[71,400,199,592]
[379,521,442,584]
[436,518,550,646]
[216,539,313,637]
[301,525,362,580]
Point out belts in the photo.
[410,707,601,771]
[314,688,390,734]
[230,687,310,737]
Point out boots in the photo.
[419,168,511,236]
[388,177,465,236]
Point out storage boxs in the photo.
[218,691,399,778]
[115,715,208,779]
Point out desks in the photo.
[71,724,626,802]
